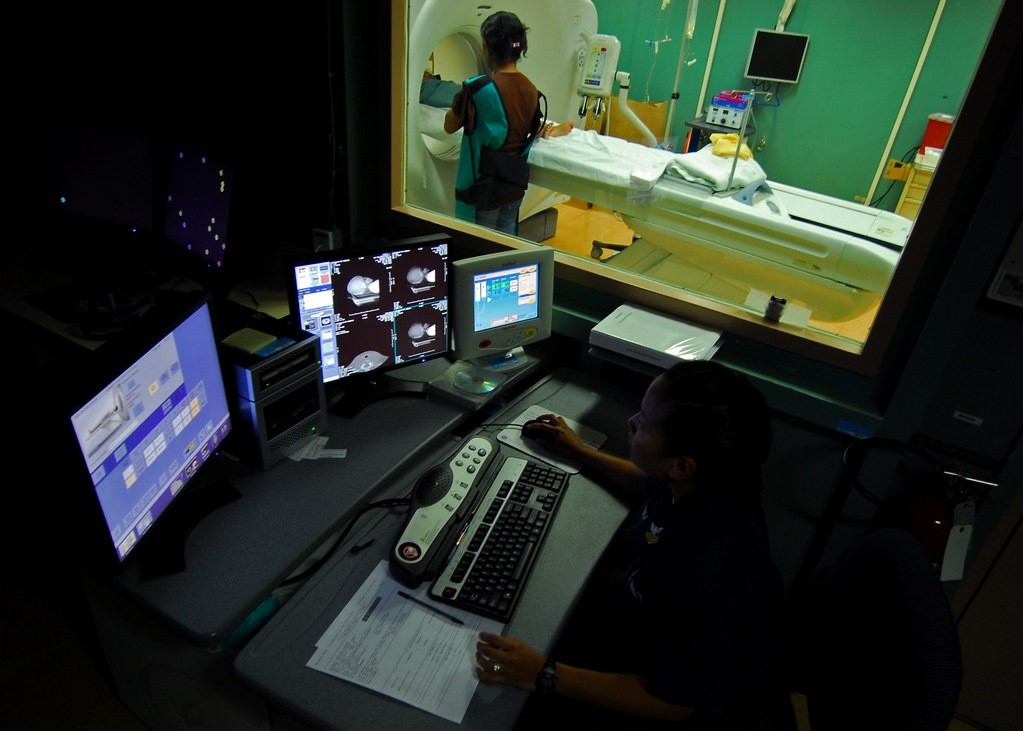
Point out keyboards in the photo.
[426,452,571,624]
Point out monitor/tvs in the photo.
[285,234,450,411]
[743,28,810,83]
[451,246,554,374]
[50,293,238,581]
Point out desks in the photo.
[70,361,640,731]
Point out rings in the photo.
[491,662,504,674]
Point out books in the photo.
[589,301,726,370]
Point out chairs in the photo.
[683,528,965,731]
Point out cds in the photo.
[452,367,498,393]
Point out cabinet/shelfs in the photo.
[895,165,934,221]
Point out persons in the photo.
[444,11,538,236]
[474,361,801,731]
[419,71,574,144]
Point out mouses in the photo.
[522,419,552,445]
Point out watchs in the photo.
[535,656,558,696]
